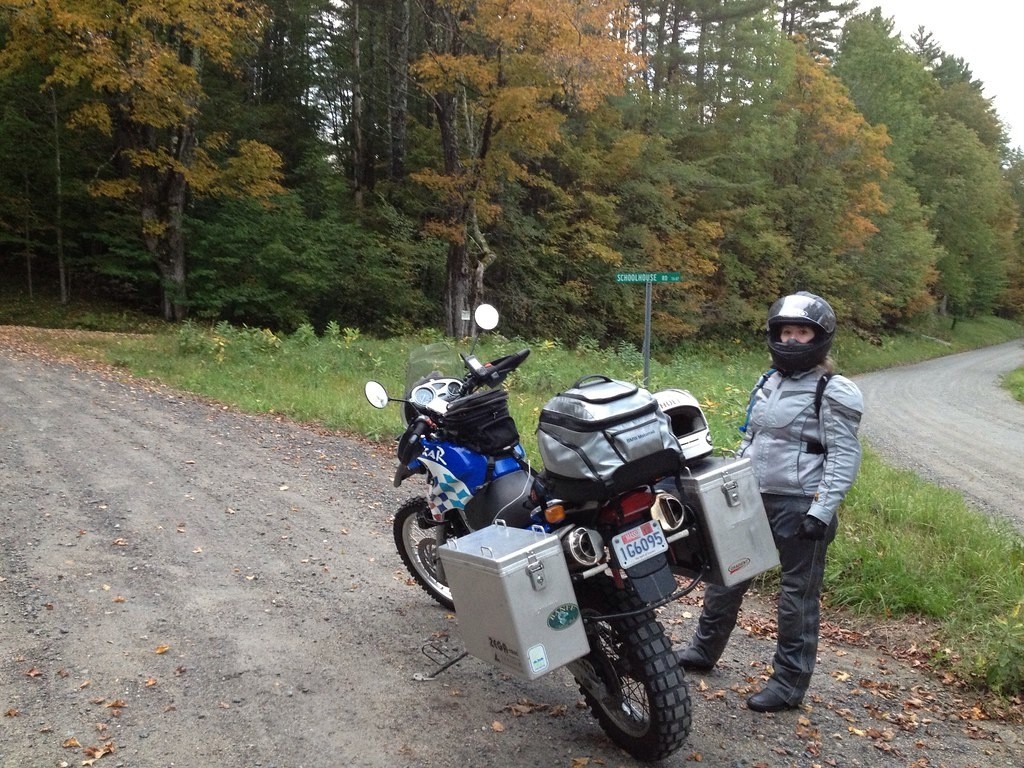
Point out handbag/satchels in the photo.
[535,374,686,504]
[443,389,519,456]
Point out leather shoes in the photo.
[673,649,714,671]
[747,688,798,712]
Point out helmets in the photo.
[767,291,836,374]
[652,389,714,463]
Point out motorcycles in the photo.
[359,303,781,764]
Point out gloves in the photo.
[798,516,827,542]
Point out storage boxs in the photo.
[655,448,783,587]
[438,518,592,681]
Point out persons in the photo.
[675,292,864,713]
